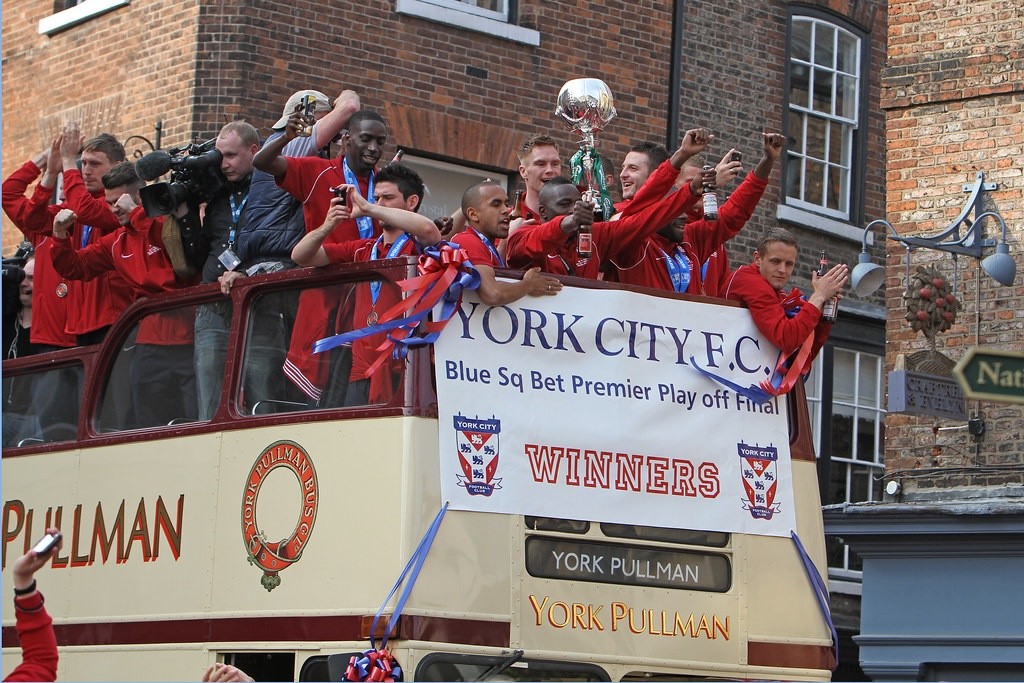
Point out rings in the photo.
[548,285,551,291]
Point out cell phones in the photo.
[334,189,347,207]
[32,533,62,555]
[730,151,742,176]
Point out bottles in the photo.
[817,249,827,277]
[577,192,592,259]
[822,291,838,324]
[703,165,718,221]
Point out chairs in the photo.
[16,397,310,446]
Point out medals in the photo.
[56,283,68,297]
[367,311,378,326]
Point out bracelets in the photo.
[14,578,38,596]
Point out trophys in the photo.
[555,78,617,222]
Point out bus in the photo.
[1,257,832,681]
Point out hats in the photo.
[272,89,331,129]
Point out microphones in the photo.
[135,151,171,182]
[102,161,139,190]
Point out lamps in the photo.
[850,171,1016,300]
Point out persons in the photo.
[202,662,254,682]
[1,526,64,682]
[2,89,848,447]
